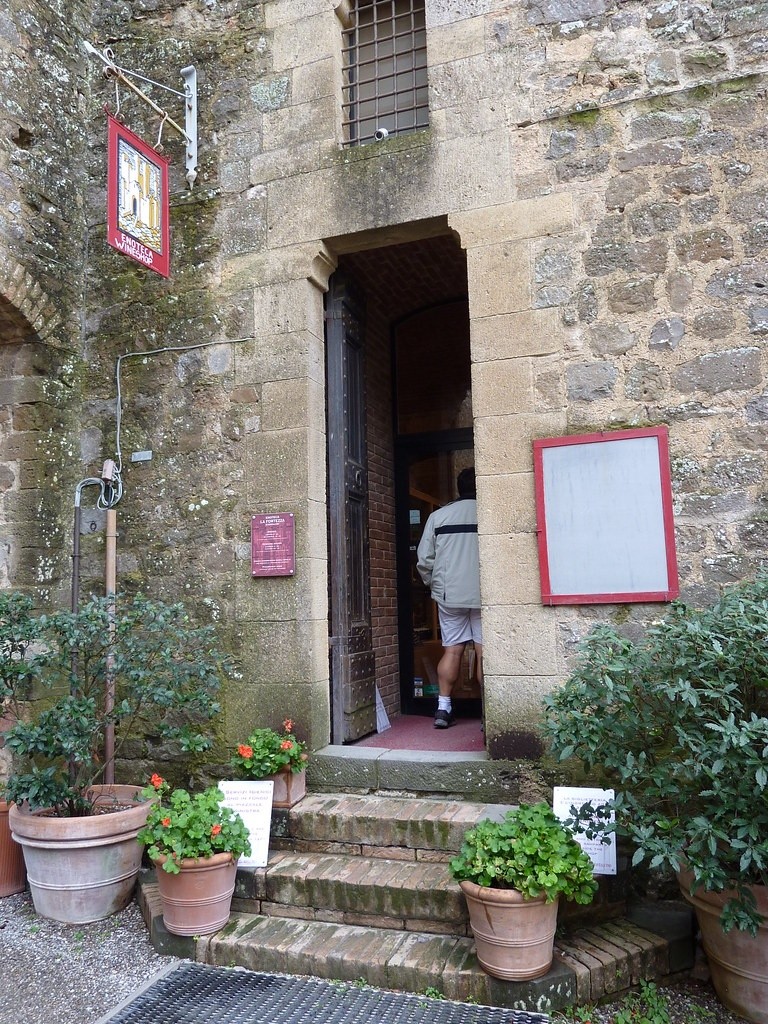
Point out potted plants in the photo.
[0,590,236,924]
[448,802,598,982]
[537,564,768,1024]
[0,782,26,898]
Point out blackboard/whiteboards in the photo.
[218,779,274,867]
[534,429,679,605]
[553,786,617,875]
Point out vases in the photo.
[255,770,306,808]
[152,842,240,937]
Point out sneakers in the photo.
[434,708,458,728]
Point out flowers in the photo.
[232,718,309,778]
[136,774,251,874]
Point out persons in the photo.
[415,467,483,727]
